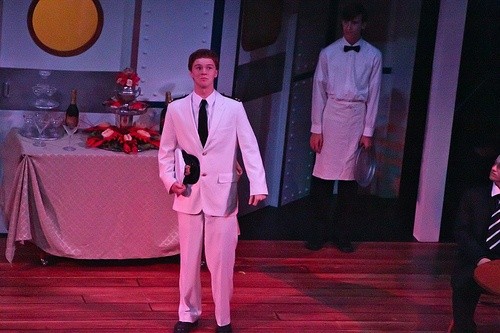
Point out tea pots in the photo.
[112,86,141,103]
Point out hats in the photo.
[175,148,200,186]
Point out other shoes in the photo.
[336,240,354,252]
[307,233,320,249]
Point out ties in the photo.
[198,99,208,148]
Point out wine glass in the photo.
[62,122,80,151]
[31,71,57,106]
[32,116,52,147]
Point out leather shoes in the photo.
[172,319,199,333]
[216,323,233,333]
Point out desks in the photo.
[2,127,242,266]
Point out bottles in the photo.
[66,89,79,133]
[160,91,171,134]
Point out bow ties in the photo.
[344,45,360,52]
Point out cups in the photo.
[20,112,66,139]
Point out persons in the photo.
[448,148,500,333]
[305,5,383,253]
[157,48,269,333]
